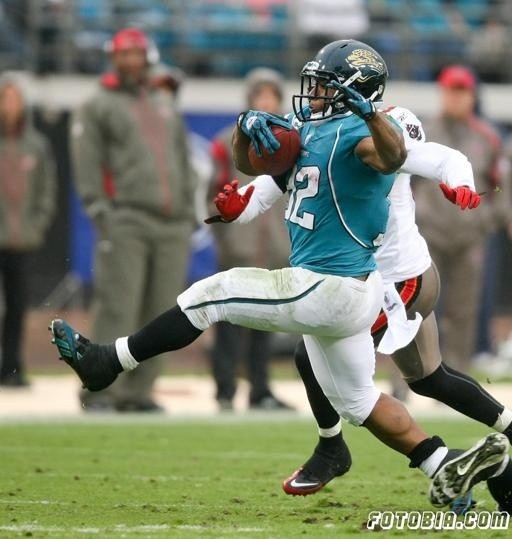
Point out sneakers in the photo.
[427,426,511,510]
[281,442,357,497]
[110,391,299,413]
[428,443,479,521]
[49,314,125,392]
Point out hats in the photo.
[437,60,481,92]
[109,27,153,54]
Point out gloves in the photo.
[239,105,297,158]
[212,178,256,224]
[319,74,380,124]
[437,180,484,212]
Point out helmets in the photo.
[307,35,392,107]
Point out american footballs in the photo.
[249,125,300,175]
